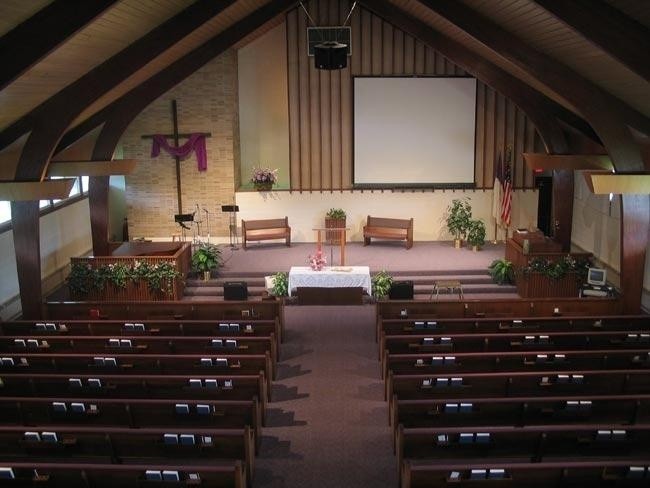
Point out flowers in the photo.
[66,261,187,298]
[528,254,592,284]
[310,249,327,271]
[249,165,279,187]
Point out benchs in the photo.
[362,215,414,250]
[241,216,292,251]
[373,296,650,486]
[1,297,285,488]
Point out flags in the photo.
[500,152,512,228]
[492,153,502,226]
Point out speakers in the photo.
[390,280,414,300]
[224,281,247,301]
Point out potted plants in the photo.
[325,209,346,245]
[191,248,220,281]
[444,197,487,251]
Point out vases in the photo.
[254,183,272,190]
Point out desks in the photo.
[429,279,465,301]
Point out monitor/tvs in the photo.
[588,267,607,290]
